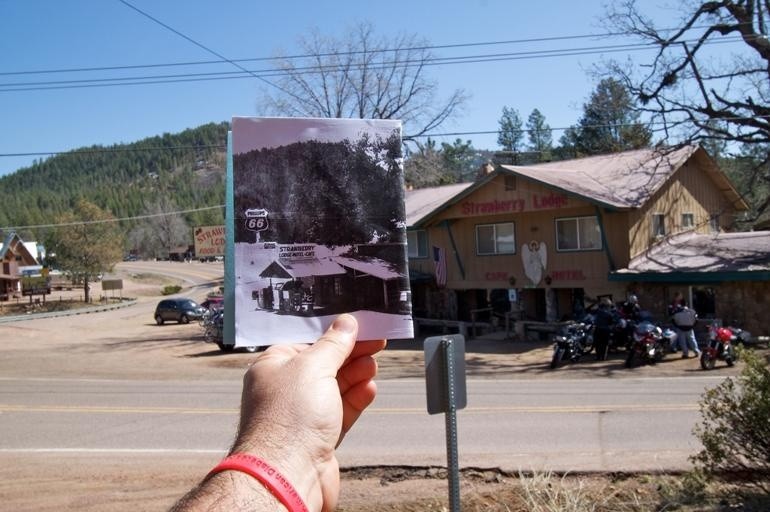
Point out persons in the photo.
[669,304,701,359]
[626,295,641,315]
[667,290,687,318]
[163,310,392,512]
[593,303,616,362]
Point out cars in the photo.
[154,297,210,325]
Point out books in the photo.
[218,112,417,349]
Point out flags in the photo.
[431,244,447,289]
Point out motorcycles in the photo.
[549,315,751,371]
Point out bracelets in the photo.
[201,452,311,512]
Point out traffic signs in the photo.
[245,218,269,232]
